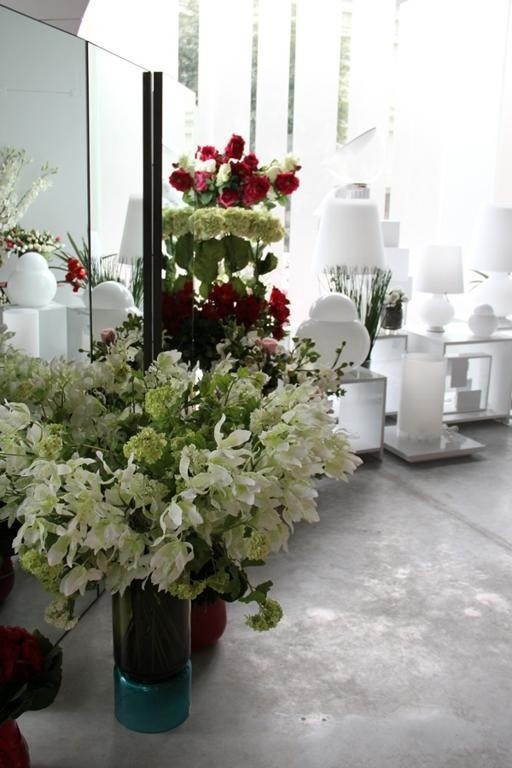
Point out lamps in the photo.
[416,246,464,332]
[322,194,385,277]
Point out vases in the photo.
[380,307,403,329]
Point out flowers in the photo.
[378,288,409,308]
[0,129,362,768]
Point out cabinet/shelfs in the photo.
[326,325,512,462]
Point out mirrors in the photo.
[1,3,195,369]
